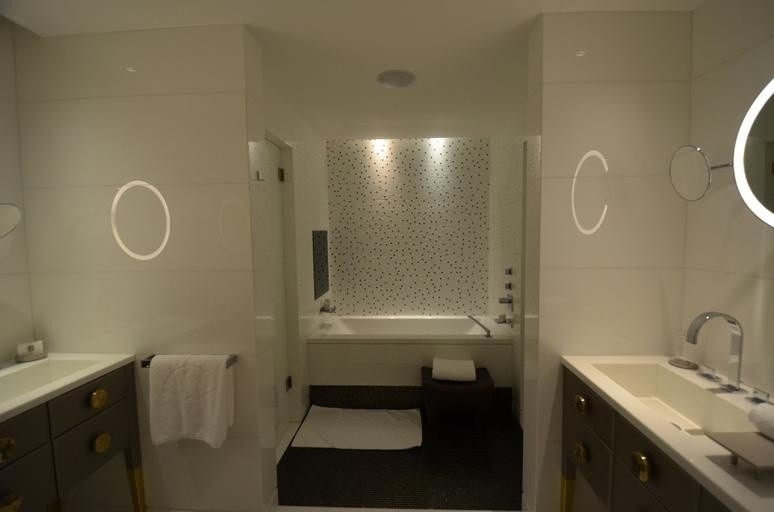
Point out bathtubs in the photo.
[305,315,514,388]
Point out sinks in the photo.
[592,361,758,438]
[0,352,137,422]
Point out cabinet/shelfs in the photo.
[0,362,147,512]
[562,367,727,511]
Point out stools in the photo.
[421,367,494,429]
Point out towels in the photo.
[430,358,476,382]
[147,355,236,447]
[747,403,774,442]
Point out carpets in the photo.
[290,404,423,451]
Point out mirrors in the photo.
[669,146,712,202]
[734,79,774,224]
[0,201,22,237]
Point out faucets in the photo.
[498,295,513,310]
[686,311,743,390]
